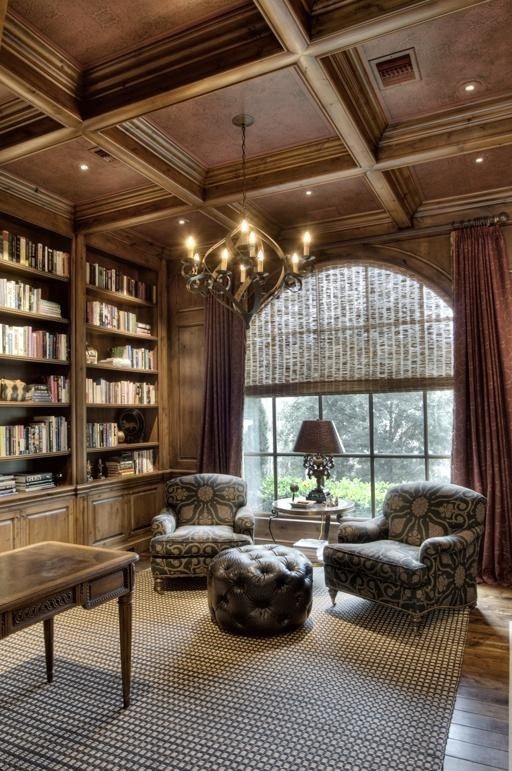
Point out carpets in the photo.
[0,563,471,770]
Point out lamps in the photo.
[292,418,345,503]
[180,113,319,330]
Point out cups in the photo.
[330,496,339,507]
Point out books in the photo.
[1,471,55,496]
[106,449,153,477]
[86,343,155,404]
[87,422,117,448]
[292,538,329,563]
[86,263,156,337]
[0,416,67,456]
[1,228,70,316]
[0,324,70,403]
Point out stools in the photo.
[209,544,314,636]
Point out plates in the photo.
[116,409,145,441]
[289,501,317,508]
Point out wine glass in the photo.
[290,481,299,502]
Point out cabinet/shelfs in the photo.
[0,191,164,559]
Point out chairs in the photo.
[150,473,254,591]
[323,482,487,634]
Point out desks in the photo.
[0,540,140,708]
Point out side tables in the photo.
[268,497,354,544]
